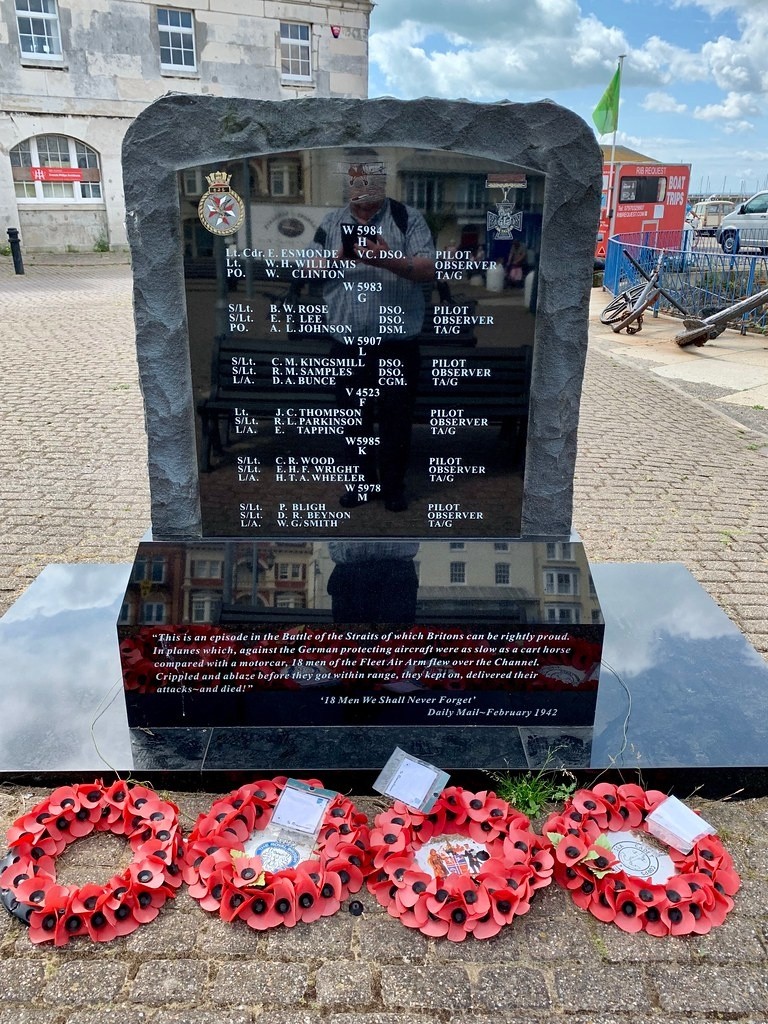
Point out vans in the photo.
[716,191,768,252]
[686,201,742,232]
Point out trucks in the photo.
[593,161,691,271]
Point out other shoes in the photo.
[384,486,407,511]
[341,487,373,505]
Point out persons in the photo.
[309,147,439,516]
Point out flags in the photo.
[591,65,623,149]
